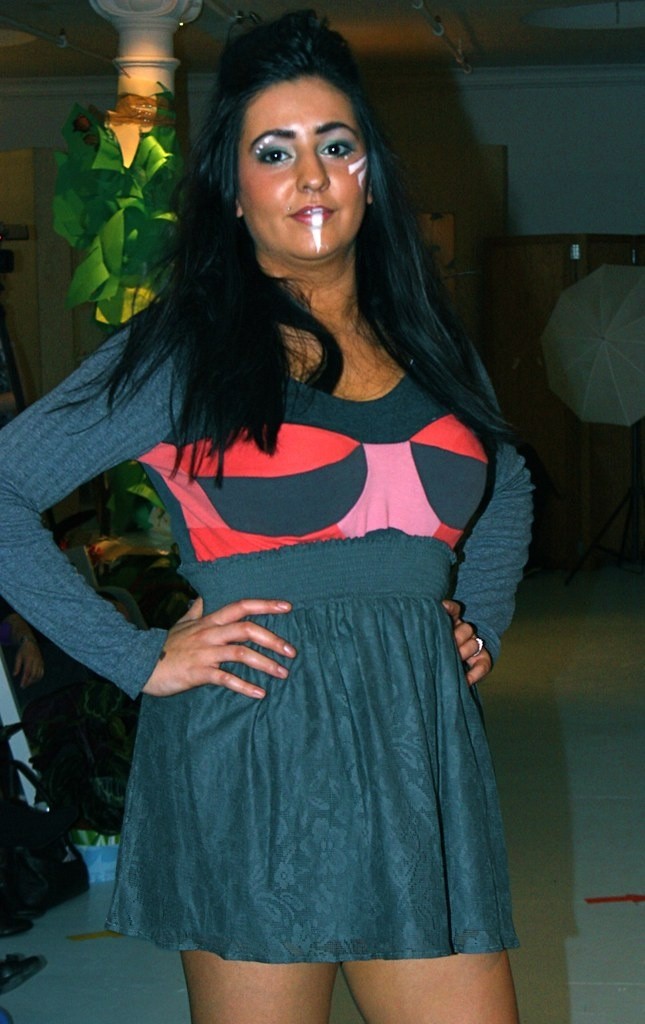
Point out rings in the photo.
[472,635,483,657]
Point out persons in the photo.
[0,595,43,688]
[0,19,535,1024]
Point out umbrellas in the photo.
[540,263,645,427]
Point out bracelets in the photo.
[18,635,38,645]
